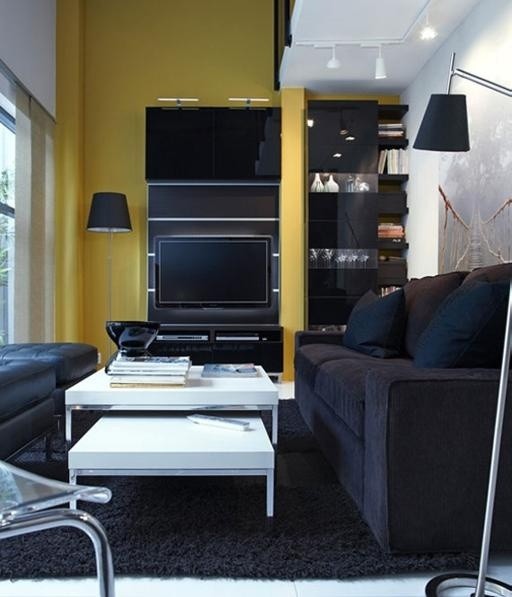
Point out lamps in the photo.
[411,2,512,156]
[85,191,134,362]
[157,97,272,110]
[326,44,388,80]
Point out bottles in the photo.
[309,173,340,193]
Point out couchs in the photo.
[295,268,512,556]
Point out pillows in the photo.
[339,261,512,368]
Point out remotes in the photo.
[187,414,250,432]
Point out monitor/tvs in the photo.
[144,106,281,186]
[155,234,271,308]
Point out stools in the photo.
[0,341,119,597]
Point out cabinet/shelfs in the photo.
[307,98,410,327]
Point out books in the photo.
[199,361,260,378]
[378,283,401,298]
[375,121,412,243]
[107,354,194,388]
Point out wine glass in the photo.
[309,248,370,270]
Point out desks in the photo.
[62,361,282,519]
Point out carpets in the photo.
[0,394,484,581]
